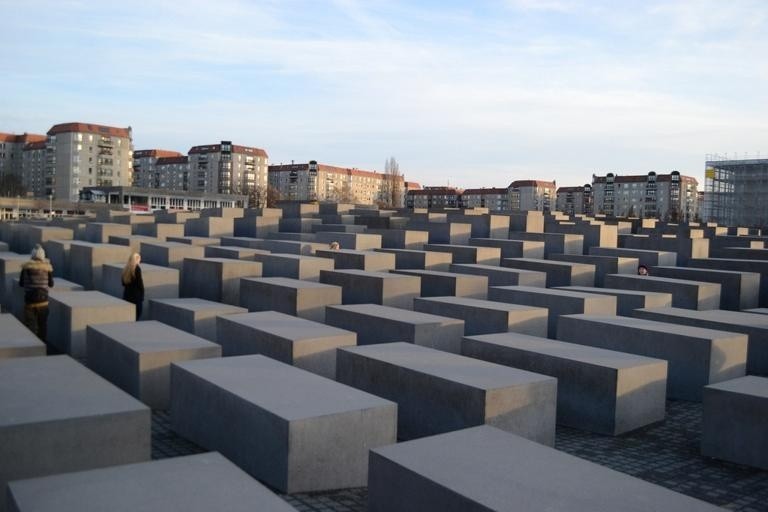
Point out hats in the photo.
[30,243,46,261]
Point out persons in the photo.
[19,244,55,344]
[121,252,144,321]
[329,241,340,250]
[638,264,648,276]
